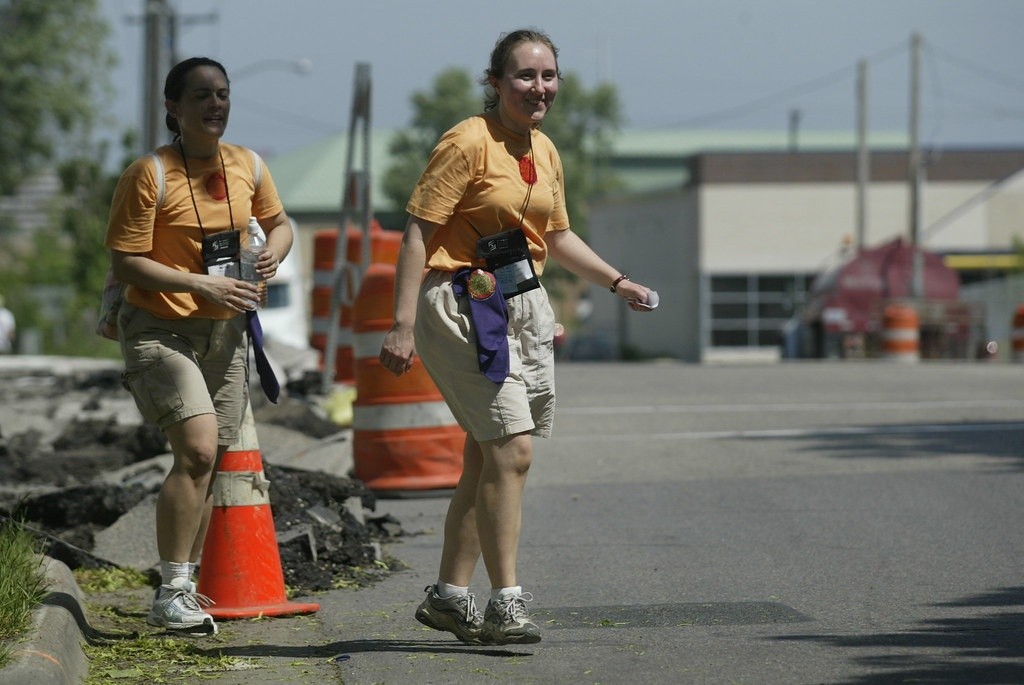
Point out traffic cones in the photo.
[196,395,320,618]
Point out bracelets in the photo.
[610,274,628,293]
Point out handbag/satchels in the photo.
[202,229,241,282]
[478,227,540,300]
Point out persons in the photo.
[103,57,294,636]
[381,31,659,645]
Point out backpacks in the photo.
[96,146,261,343]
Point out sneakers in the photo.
[146,586,218,638]
[414,585,498,645]
[478,594,542,646]
